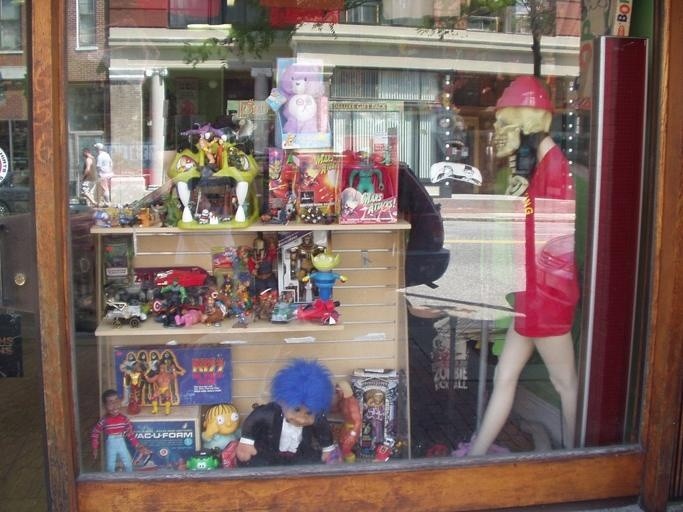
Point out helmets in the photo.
[492,76,556,117]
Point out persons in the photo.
[88,121,406,476]
[78,148,99,208]
[91,141,116,209]
[449,71,580,459]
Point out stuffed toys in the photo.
[268,59,324,134]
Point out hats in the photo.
[92,142,103,149]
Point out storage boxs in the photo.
[118,403,203,471]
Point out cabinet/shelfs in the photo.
[85,206,411,467]
